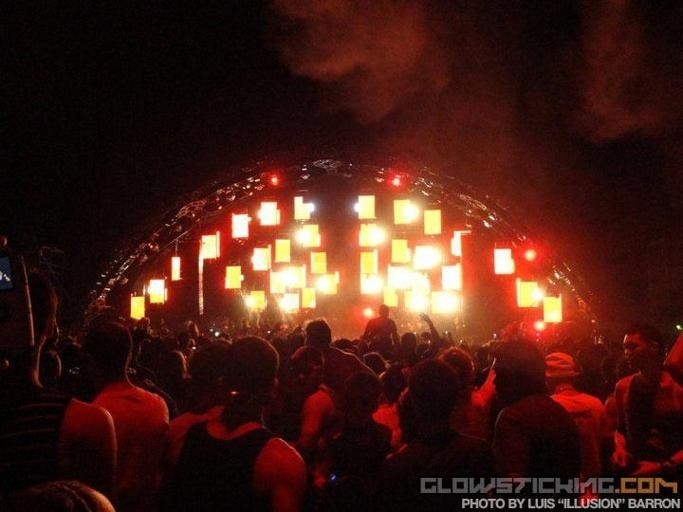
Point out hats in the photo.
[545,351,577,378]
